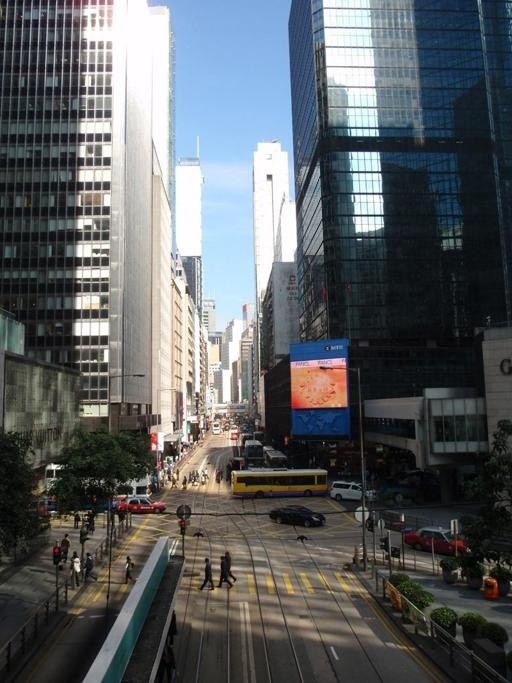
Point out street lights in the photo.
[269,504,326,528]
[329,481,376,502]
[108,373,145,435]
[319,366,368,572]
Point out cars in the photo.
[115,496,166,514]
[403,526,472,558]
[370,507,406,531]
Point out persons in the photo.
[222,552,236,582]
[70,552,81,586]
[216,556,233,588]
[74,496,127,535]
[60,534,71,564]
[124,556,136,585]
[199,557,214,590]
[166,462,234,490]
[82,553,97,583]
[154,610,179,683]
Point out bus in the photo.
[231,466,327,498]
[211,422,220,435]
[230,424,238,440]
[263,445,288,471]
[244,439,263,471]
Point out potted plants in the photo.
[387,556,511,650]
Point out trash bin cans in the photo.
[484,578,499,601]
